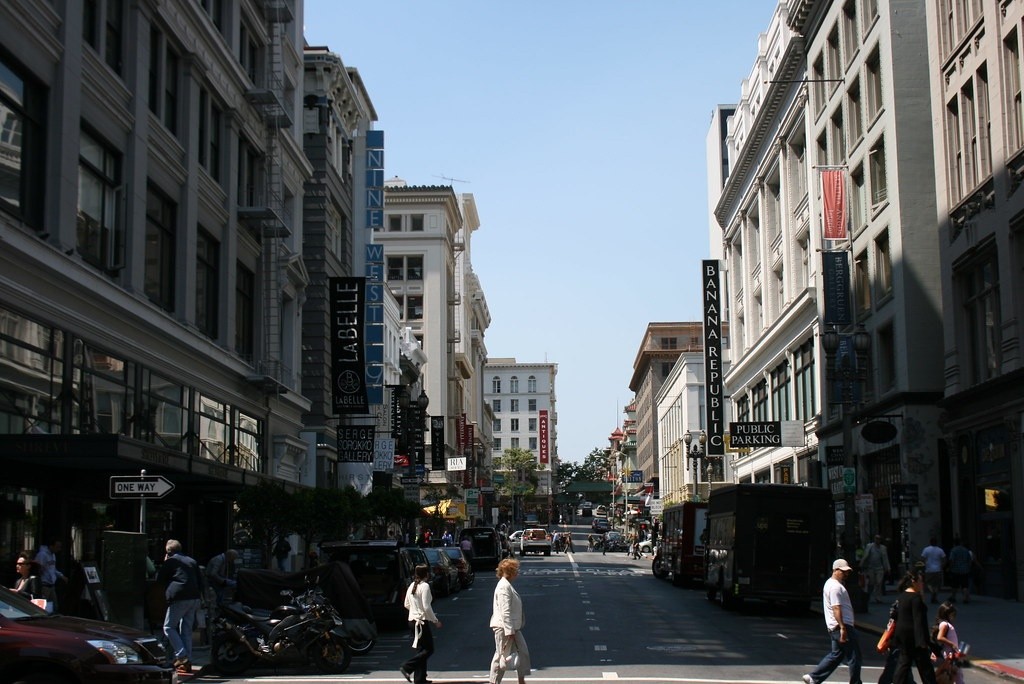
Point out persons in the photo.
[366,526,454,548]
[968,549,984,601]
[877,569,967,684]
[802,558,864,684]
[34,538,69,613]
[488,558,526,684]
[588,533,643,559]
[919,534,948,605]
[884,538,895,585]
[9,551,42,600]
[947,538,972,603]
[397,563,444,684]
[206,550,239,605]
[552,529,575,553]
[459,535,474,564]
[155,539,205,674]
[858,535,891,596]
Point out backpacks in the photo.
[930,621,950,666]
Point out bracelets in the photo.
[840,628,846,629]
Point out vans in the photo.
[459,527,502,570]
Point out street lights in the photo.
[600,448,628,533]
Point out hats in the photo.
[832,559,852,571]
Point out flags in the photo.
[821,251,853,327]
[329,276,371,414]
[826,333,863,404]
[431,416,446,471]
[822,169,851,242]
[455,413,476,489]
[408,401,425,479]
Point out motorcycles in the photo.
[211,572,375,677]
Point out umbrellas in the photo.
[52,548,85,608]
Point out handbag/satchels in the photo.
[500,634,520,670]
[875,620,896,653]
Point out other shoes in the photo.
[415,680,432,684]
[400,667,412,682]
[174,657,193,674]
[802,674,815,684]
[931,592,936,604]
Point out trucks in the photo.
[652,501,709,581]
[704,483,837,616]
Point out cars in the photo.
[509,531,524,551]
[442,547,475,586]
[582,501,630,552]
[316,541,436,631]
[421,546,460,599]
[0,585,172,684]
[629,539,652,553]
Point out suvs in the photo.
[519,528,553,557]
